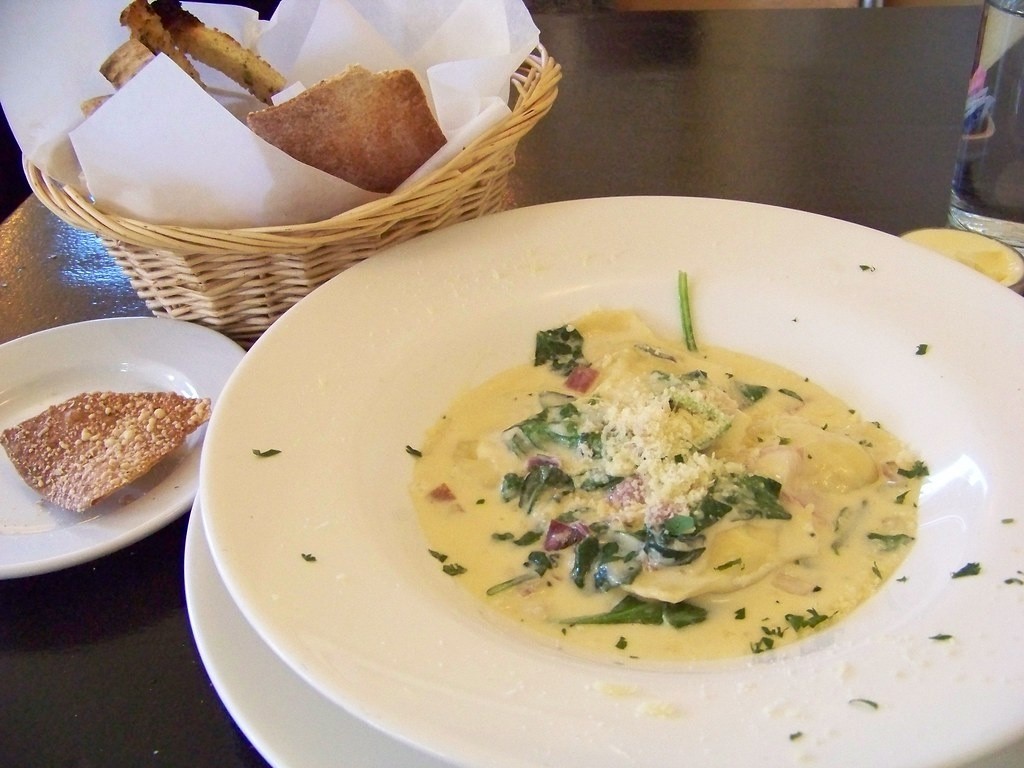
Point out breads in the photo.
[80,37,154,118]
[247,64,450,196]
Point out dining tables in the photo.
[0,4,1024,768]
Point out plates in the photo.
[0,314,248,579]
[184,491,448,768]
[197,194,1022,768]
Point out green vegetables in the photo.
[253,268,986,655]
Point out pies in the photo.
[0,390,215,511]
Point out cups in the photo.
[941,0,1024,259]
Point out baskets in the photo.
[23,26,560,351]
